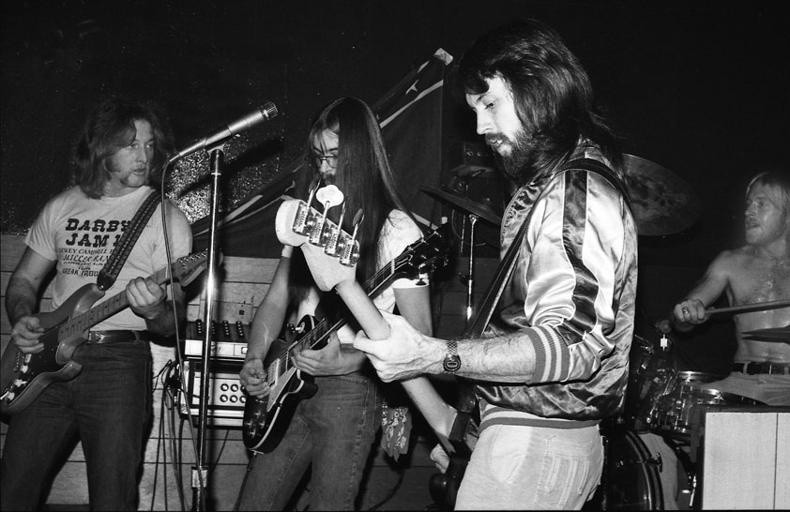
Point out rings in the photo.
[683,308,689,314]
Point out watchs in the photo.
[443,337,461,376]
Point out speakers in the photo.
[693,404,790,510]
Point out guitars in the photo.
[1,248,223,411]
[243,228,448,454]
[275,184,481,511]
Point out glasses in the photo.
[314,153,338,168]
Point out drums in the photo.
[635,365,711,437]
[676,389,769,468]
[583,427,694,510]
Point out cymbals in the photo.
[741,326,790,343]
[622,153,703,234]
[415,187,504,232]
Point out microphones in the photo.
[170,97,281,163]
[457,169,484,191]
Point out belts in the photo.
[84,331,147,345]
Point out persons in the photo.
[353,14,640,512]
[0,97,192,512]
[236,94,433,512]
[673,172,790,407]
